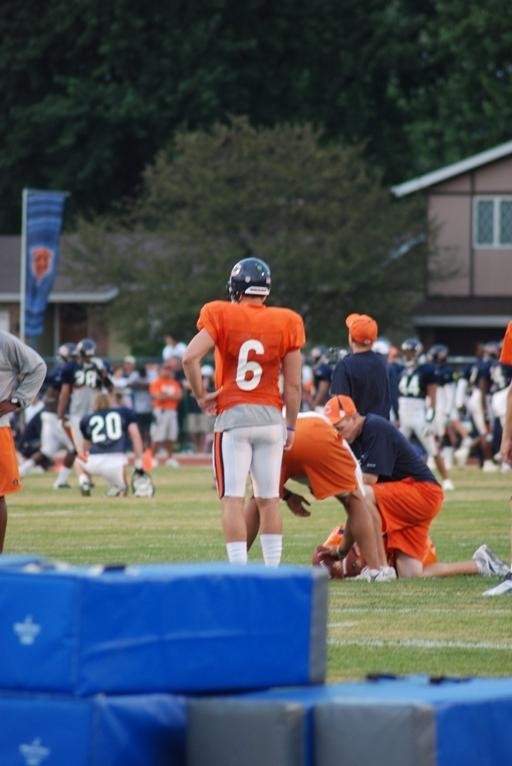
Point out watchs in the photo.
[10,398,21,407]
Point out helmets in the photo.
[71,338,96,357]
[227,257,271,302]
[430,345,448,362]
[402,338,426,367]
[58,342,76,362]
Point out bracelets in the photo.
[336,547,347,559]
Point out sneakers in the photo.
[482,459,511,473]
[474,545,512,580]
[345,569,385,582]
[380,567,397,581]
[80,482,89,496]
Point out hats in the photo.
[345,313,378,346]
[322,395,356,424]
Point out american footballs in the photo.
[311,544,342,580]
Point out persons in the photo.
[318,395,510,581]
[314,520,439,578]
[0,321,47,554]
[279,314,511,491]
[182,257,300,565]
[22,333,216,496]
[242,411,382,583]
